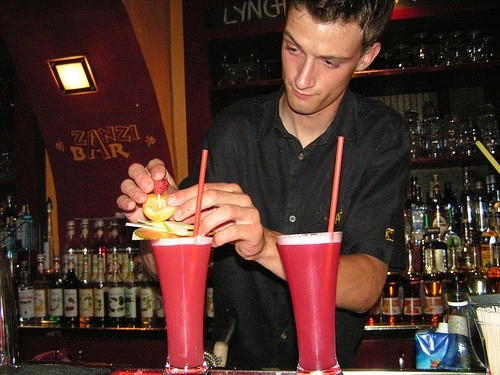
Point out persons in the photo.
[117,0,412,371]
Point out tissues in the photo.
[414,321,449,369]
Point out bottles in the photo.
[205,261,214,340]
[366,165,500,372]
[0,194,166,375]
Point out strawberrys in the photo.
[152,178,168,194]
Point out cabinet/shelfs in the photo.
[16,0,500,370]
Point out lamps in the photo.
[47,55,98,97]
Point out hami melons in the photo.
[134,228,194,240]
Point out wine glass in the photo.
[378,29,497,70]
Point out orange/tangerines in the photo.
[142,194,176,221]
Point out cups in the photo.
[276,231,344,375]
[149,236,213,375]
[405,101,500,160]
[217,51,273,86]
